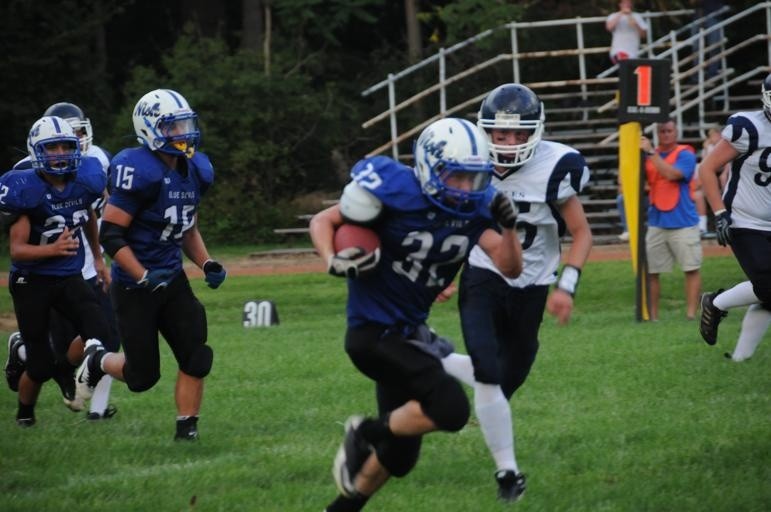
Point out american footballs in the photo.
[334,223,381,260]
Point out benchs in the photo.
[273,116,755,246]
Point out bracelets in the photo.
[558,263,581,295]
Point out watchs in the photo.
[646,149,655,155]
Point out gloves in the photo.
[328,248,381,278]
[714,211,732,247]
[488,191,517,228]
[203,259,226,289]
[138,270,176,293]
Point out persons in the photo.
[308,117,522,510]
[604,1,647,73]
[694,71,771,363]
[640,120,704,323]
[420,83,590,501]
[697,122,738,234]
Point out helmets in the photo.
[761,74,771,112]
[27,102,93,175]
[413,83,545,217]
[132,89,200,159]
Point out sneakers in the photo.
[332,415,367,498]
[700,289,728,345]
[5,332,27,392]
[494,469,525,506]
[63,338,104,412]
